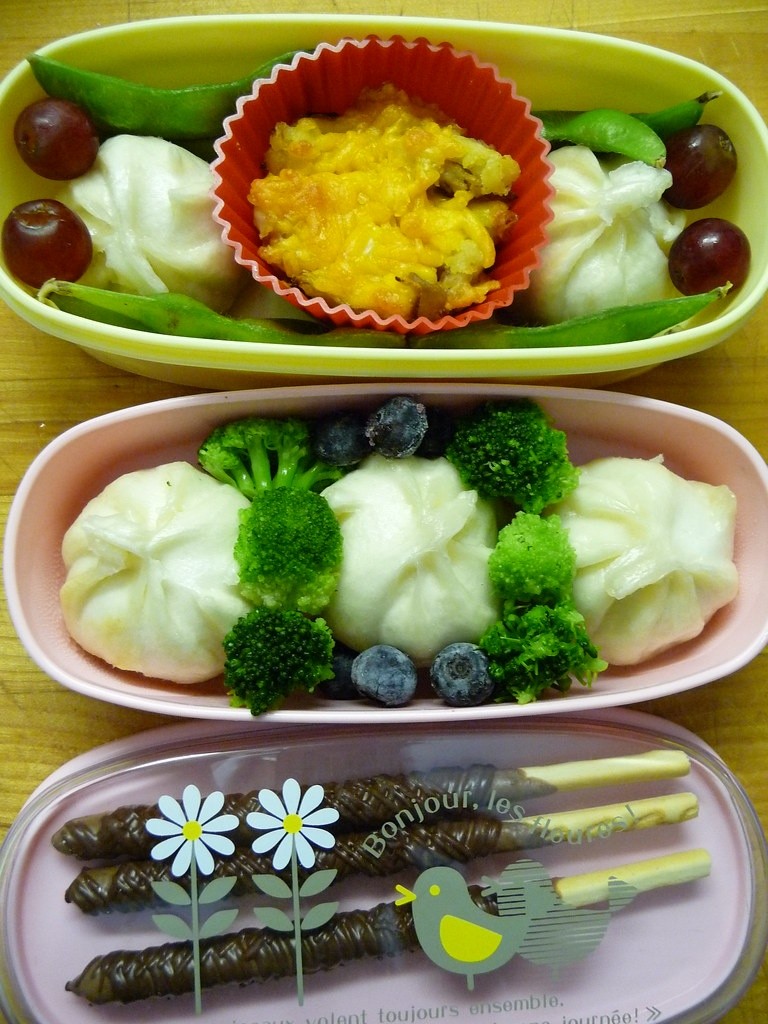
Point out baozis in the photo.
[317,455,504,663]
[60,460,257,684]
[548,455,738,667]
[47,134,249,317]
[504,143,691,326]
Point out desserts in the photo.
[210,33,560,334]
[50,746,712,1003]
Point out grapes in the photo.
[14,97,100,181]
[3,198,92,289]
[668,218,751,297]
[661,123,735,210]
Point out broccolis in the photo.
[197,396,607,716]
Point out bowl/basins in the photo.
[2,384,768,727]
[1,18,768,376]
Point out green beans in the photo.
[21,49,732,347]
